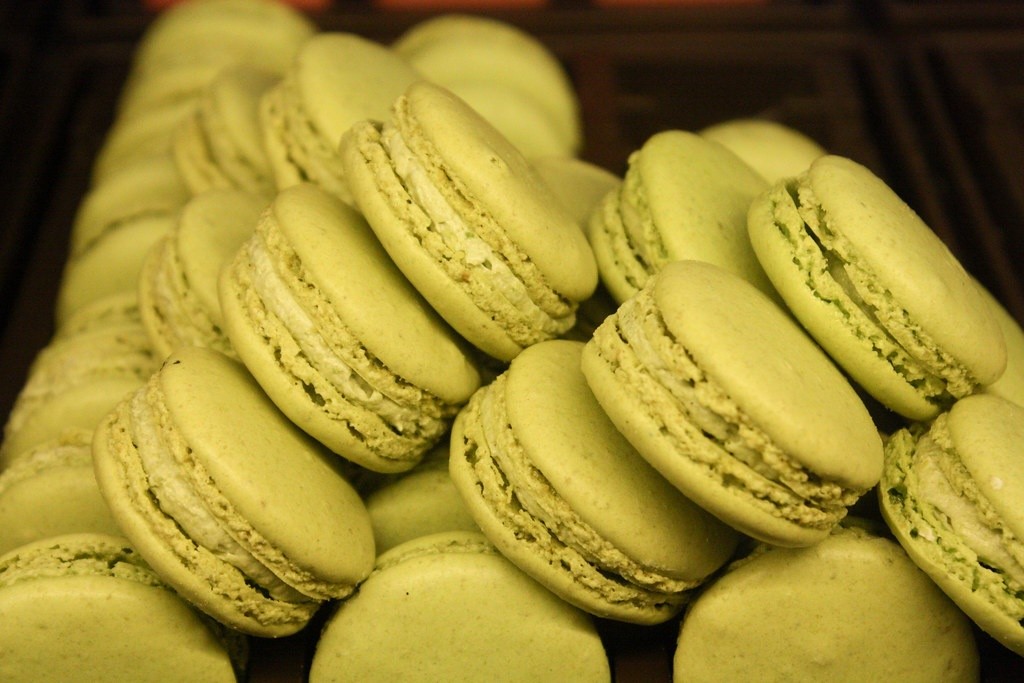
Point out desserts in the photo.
[0,0,1024,683]
